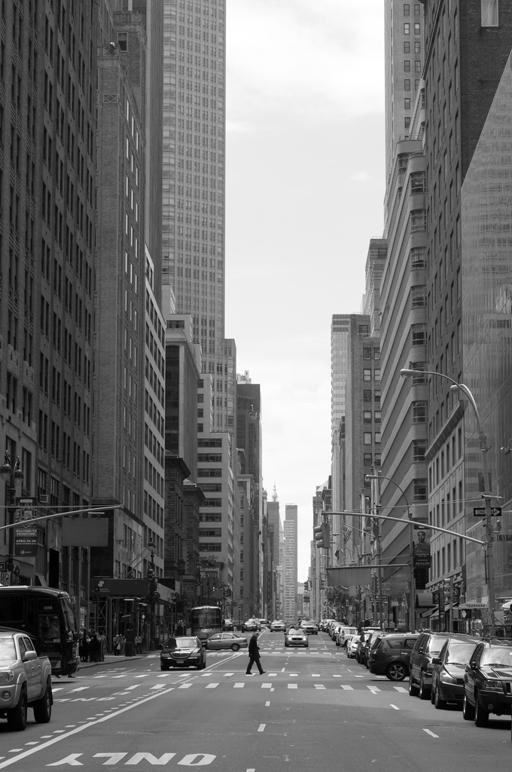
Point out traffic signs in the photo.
[12,527,37,558]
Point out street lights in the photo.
[400,369,495,635]
[0,450,24,586]
[0,505,124,529]
[366,475,416,634]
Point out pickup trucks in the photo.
[0,631,53,731]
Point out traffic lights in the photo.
[313,516,329,549]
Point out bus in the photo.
[0,583,82,673]
[191,606,225,646]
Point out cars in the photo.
[299,619,318,635]
[223,618,234,631]
[244,616,285,631]
[161,637,206,671]
[205,633,248,651]
[320,619,512,726]
[284,625,308,647]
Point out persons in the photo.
[245,631,266,676]
[80,624,142,662]
